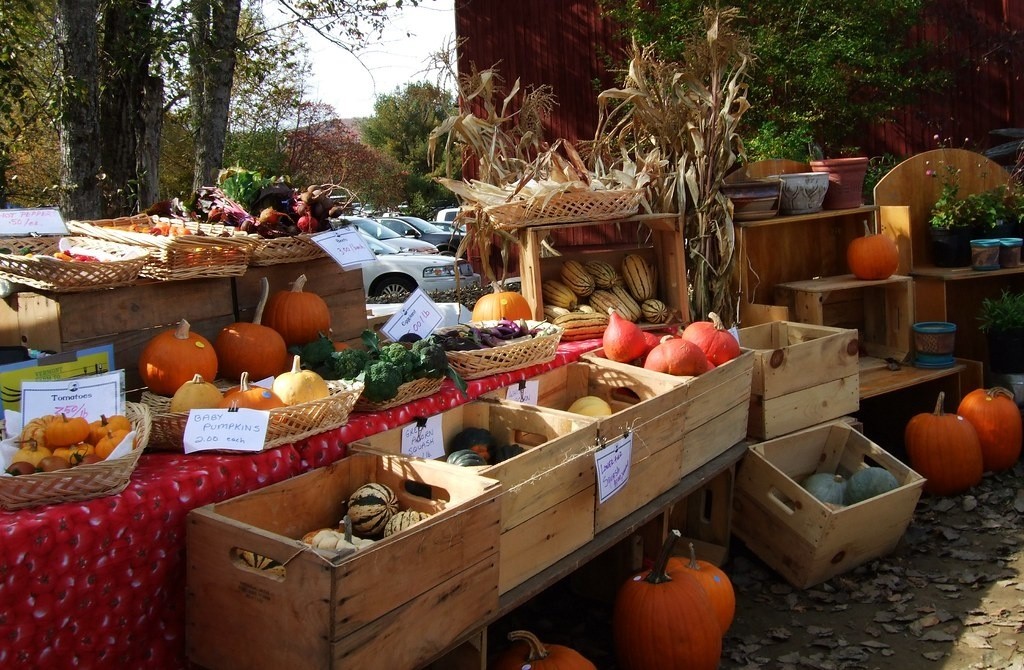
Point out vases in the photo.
[976,222,1007,240]
[990,373,1024,406]
[768,171,830,215]
[810,157,869,210]
[930,225,975,268]
[719,180,782,213]
[1007,223,1024,263]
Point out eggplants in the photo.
[431,316,557,351]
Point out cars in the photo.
[374,216,467,256]
[338,201,409,221]
[352,218,440,254]
[356,226,482,298]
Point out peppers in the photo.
[16,223,241,263]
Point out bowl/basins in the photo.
[767,171,829,215]
[719,178,781,220]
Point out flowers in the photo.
[924,134,1024,229]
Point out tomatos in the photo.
[5,447,104,476]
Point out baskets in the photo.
[66,216,257,280]
[148,379,361,451]
[354,376,442,411]
[139,391,172,412]
[249,234,330,264]
[0,237,150,291]
[0,401,151,510]
[434,321,564,379]
[484,188,647,229]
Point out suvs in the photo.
[433,208,461,222]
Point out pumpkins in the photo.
[403,427,527,499]
[905,391,983,495]
[611,528,735,670]
[798,466,899,510]
[13,273,348,462]
[489,629,597,670]
[541,252,740,420]
[234,482,435,578]
[470,281,532,322]
[845,220,899,280]
[957,386,1022,473]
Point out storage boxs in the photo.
[233,257,368,344]
[479,362,690,536]
[519,212,692,337]
[184,452,502,670]
[580,345,754,479]
[734,418,927,591]
[347,401,597,596]
[728,320,861,440]
[777,272,915,374]
[0,273,235,392]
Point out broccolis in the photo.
[289,329,448,403]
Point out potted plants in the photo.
[975,285,1024,374]
